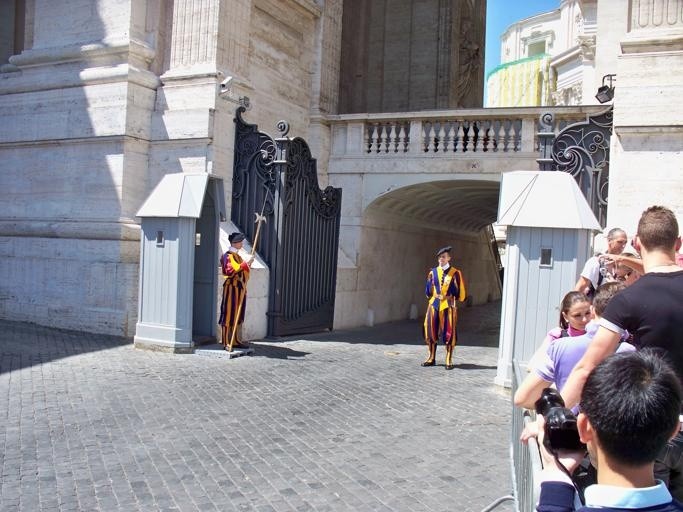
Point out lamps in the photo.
[595,84,614,104]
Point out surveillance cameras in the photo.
[220,76,233,89]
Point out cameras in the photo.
[535,388,586,452]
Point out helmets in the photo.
[431,245,451,259]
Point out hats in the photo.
[228,232,245,244]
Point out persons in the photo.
[218,232,257,353]
[419,246,468,369]
[537,350,683,512]
[514,204,683,505]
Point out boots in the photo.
[229,324,249,349]
[442,346,455,369]
[419,343,437,367]
[221,326,233,352]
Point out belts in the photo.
[433,293,452,301]
